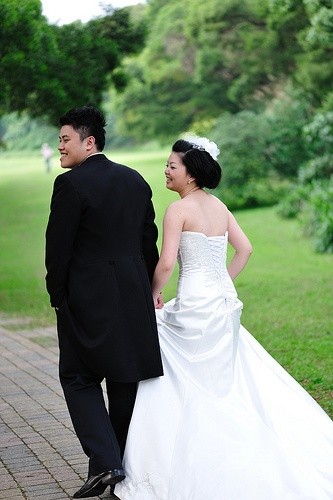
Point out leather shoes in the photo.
[72,469,126,498]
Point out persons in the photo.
[113,134,333,500]
[42,143,53,171]
[45,105,164,500]
